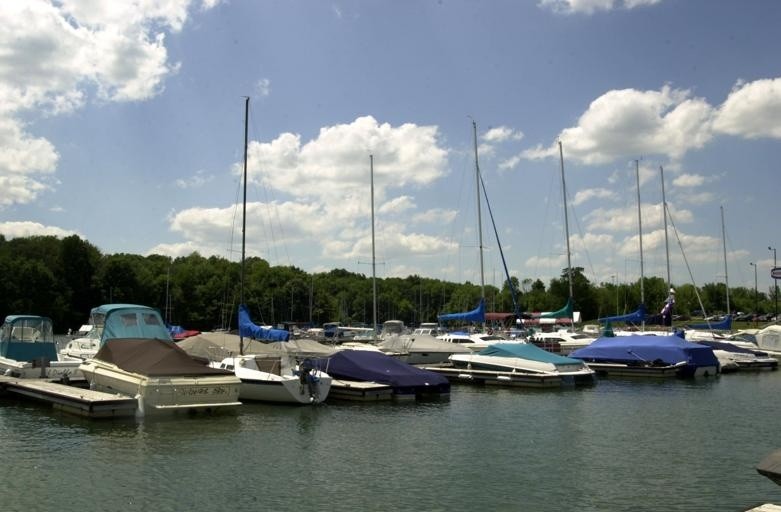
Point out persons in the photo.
[663,288,677,326]
